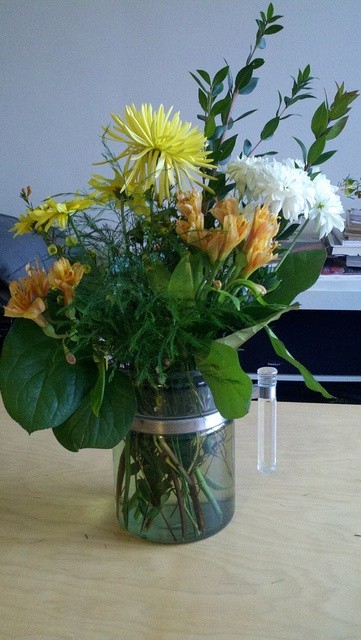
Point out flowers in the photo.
[0,3,360,452]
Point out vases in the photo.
[114,372,237,545]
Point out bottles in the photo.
[257,367,279,474]
